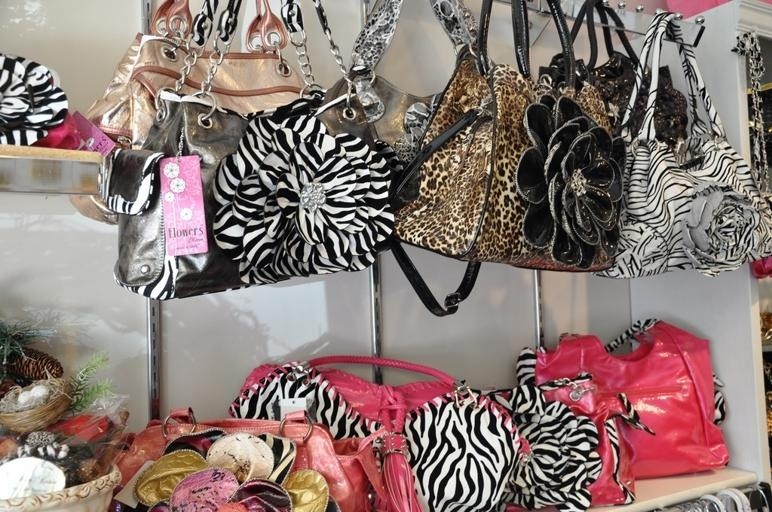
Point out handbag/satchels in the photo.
[321,0,478,164]
[536,0,690,143]
[227,354,535,512]
[589,0,772,279]
[391,0,628,317]
[514,318,731,480]
[469,371,657,512]
[67,0,308,224]
[98,0,397,302]
[111,405,388,512]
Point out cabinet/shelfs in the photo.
[0,1,770,511]
